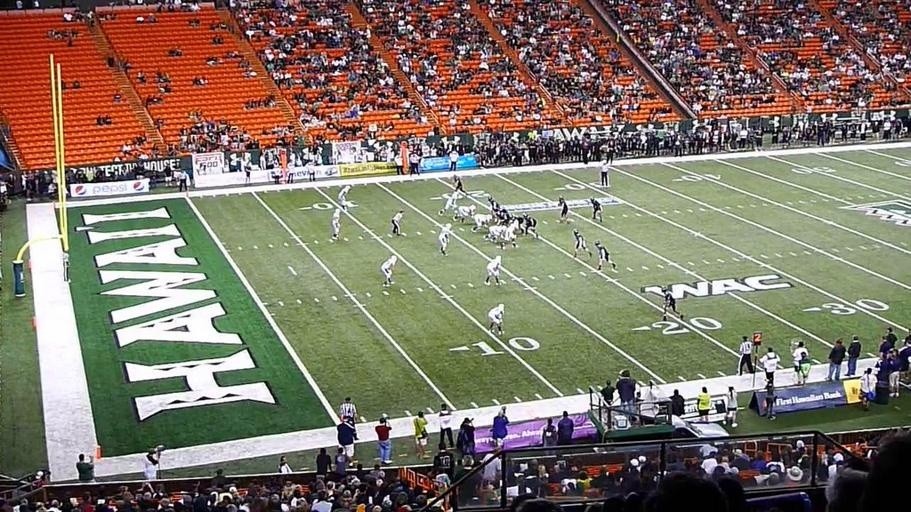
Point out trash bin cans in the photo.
[875,381,890,404]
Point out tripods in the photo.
[156,452,176,479]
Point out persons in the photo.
[660,286,685,321]
[0,324,911,512]
[0,0,910,254]
[595,243,619,273]
[381,256,398,289]
[484,256,502,286]
[487,303,506,335]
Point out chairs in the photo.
[1,1,910,173]
